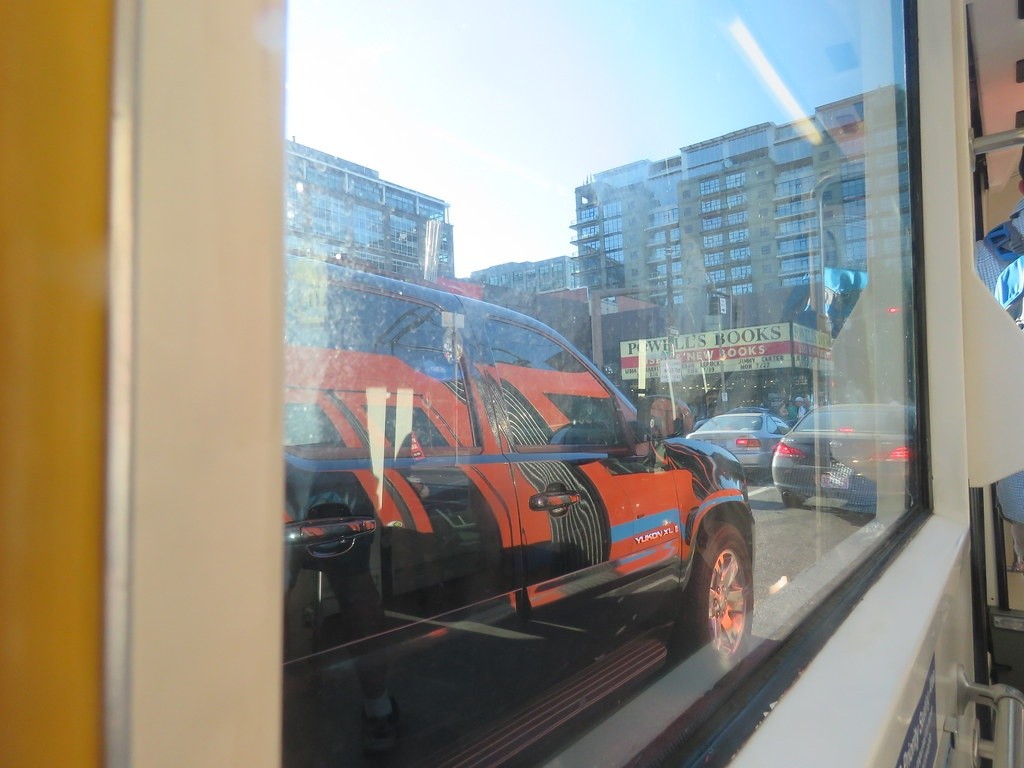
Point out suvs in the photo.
[281,254,755,768]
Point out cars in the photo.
[770,403,915,516]
[693,406,772,432]
[686,414,792,483]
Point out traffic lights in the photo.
[888,306,898,318]
[708,292,721,315]
